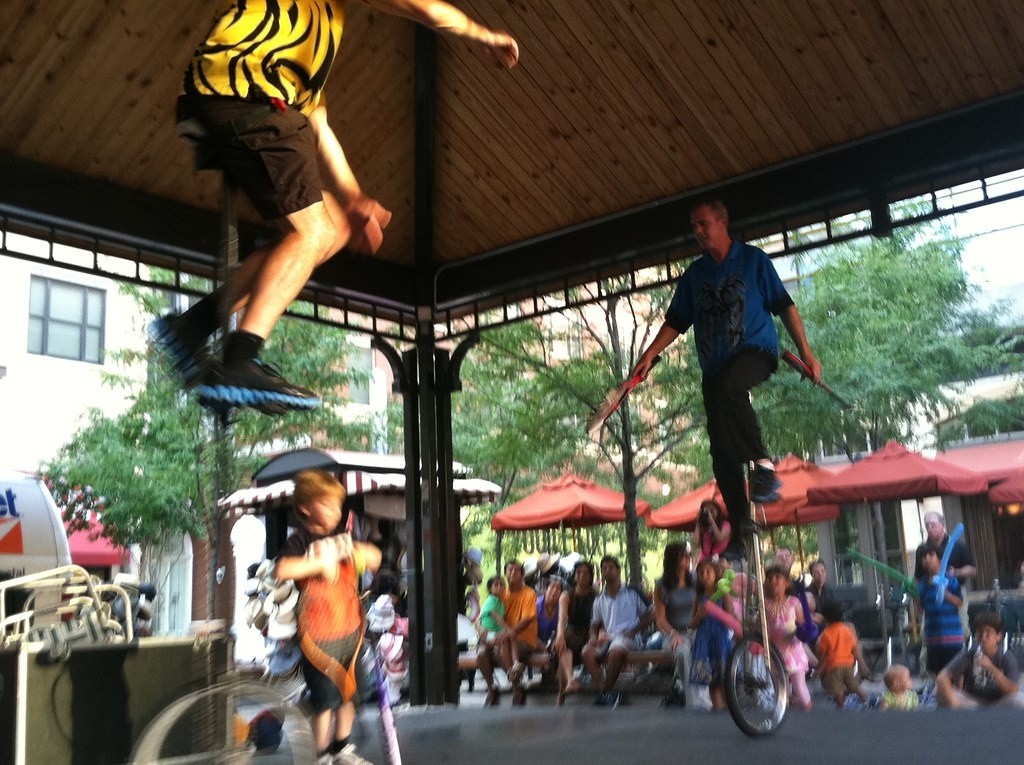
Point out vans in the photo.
[0,477,75,634]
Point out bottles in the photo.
[973,645,983,677]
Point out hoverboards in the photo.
[715,460,793,740]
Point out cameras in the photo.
[700,508,709,520]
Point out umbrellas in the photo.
[805,437,992,542]
[986,458,1024,504]
[644,451,840,574]
[490,471,651,553]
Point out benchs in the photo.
[459,648,675,671]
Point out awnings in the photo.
[933,438,1024,482]
[63,519,131,566]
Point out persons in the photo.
[274,472,382,765]
[460,500,873,712]
[879,511,1024,710]
[632,193,822,557]
[144,0,522,412]
[361,581,409,640]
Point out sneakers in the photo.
[147,313,232,415]
[313,753,334,765]
[720,524,754,563]
[333,743,373,765]
[750,464,782,503]
[199,355,321,409]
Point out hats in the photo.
[524,555,537,579]
[537,552,560,575]
[242,549,482,700]
